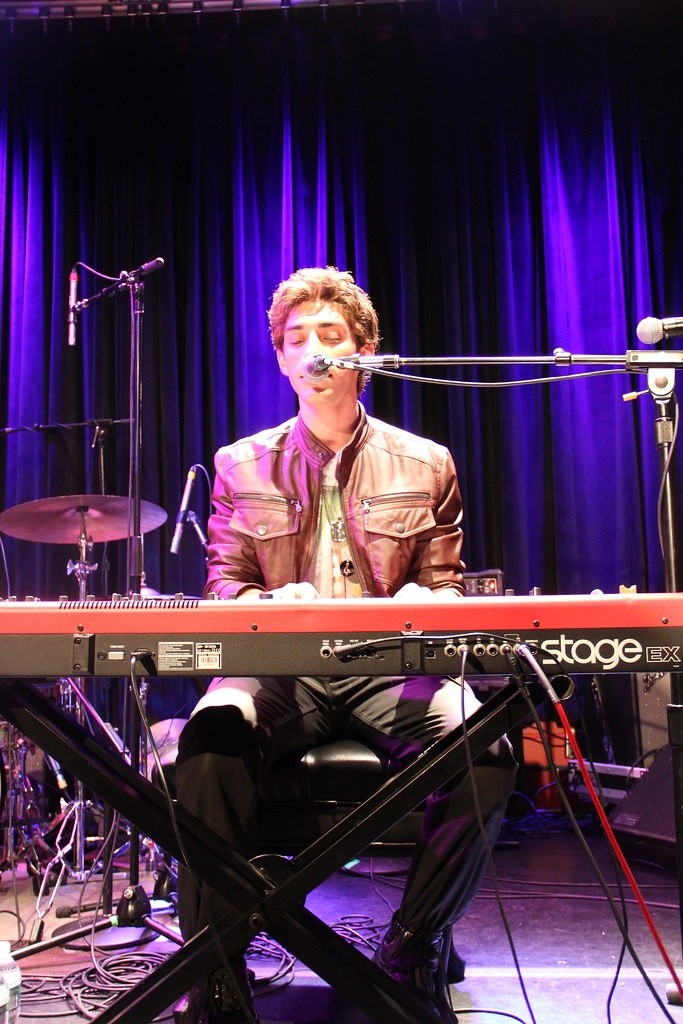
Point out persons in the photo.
[174,268,521,1024]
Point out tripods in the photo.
[8,284,191,963]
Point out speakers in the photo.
[605,741,678,877]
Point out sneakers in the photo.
[372,911,456,1024]
[186,956,260,1024]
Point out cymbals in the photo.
[123,588,203,600]
[1,494,168,543]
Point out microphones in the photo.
[636,315,683,345]
[169,465,198,555]
[302,355,332,384]
[66,269,78,346]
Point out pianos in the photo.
[1,593,683,676]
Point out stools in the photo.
[152,739,467,985]
